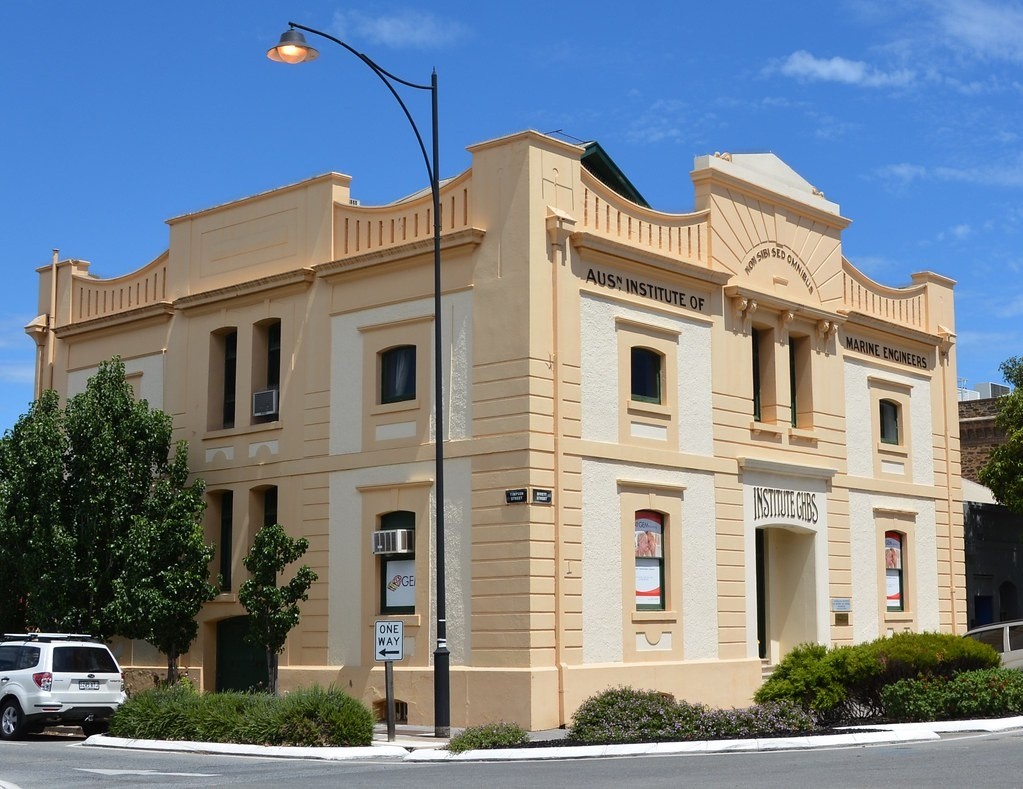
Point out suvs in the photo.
[0,632,129,740]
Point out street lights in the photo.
[264,20,451,739]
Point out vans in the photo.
[961,619,1023,674]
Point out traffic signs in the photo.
[374,620,403,661]
